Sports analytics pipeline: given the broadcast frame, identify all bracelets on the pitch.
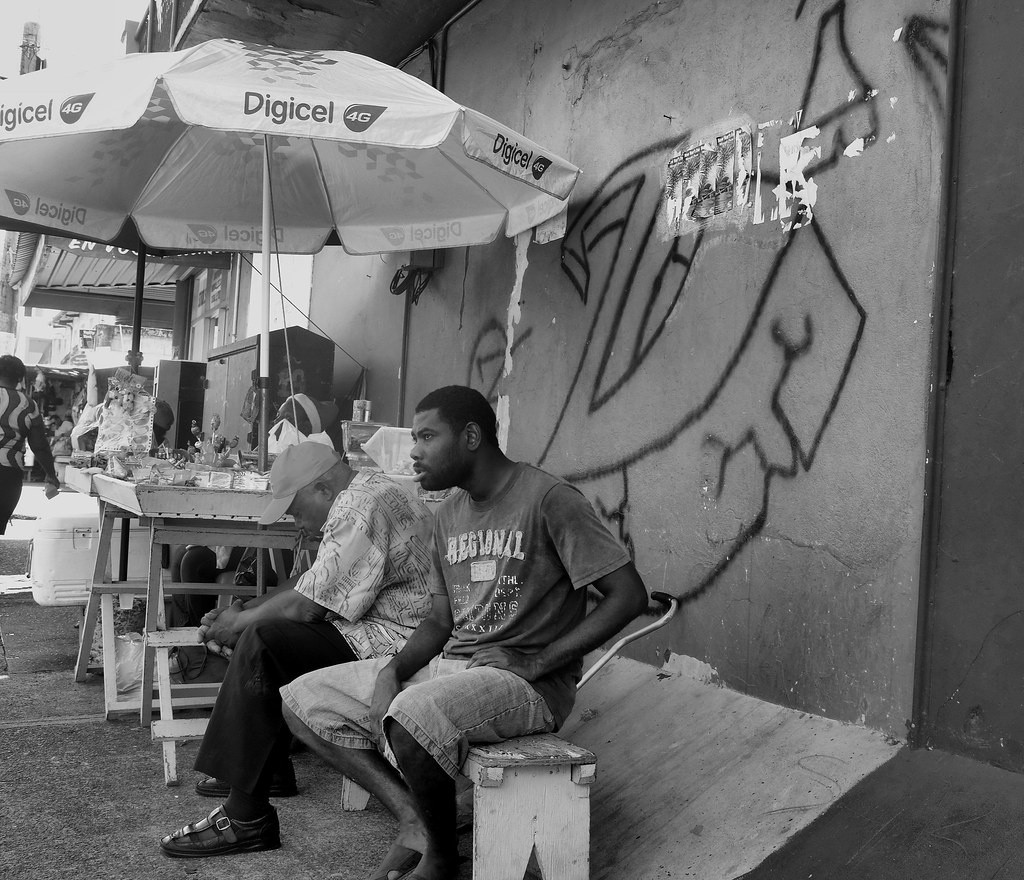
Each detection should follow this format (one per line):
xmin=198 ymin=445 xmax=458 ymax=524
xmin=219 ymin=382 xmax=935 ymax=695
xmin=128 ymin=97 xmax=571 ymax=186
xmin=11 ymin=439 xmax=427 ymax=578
xmin=239 ymin=602 xmax=246 ymax=612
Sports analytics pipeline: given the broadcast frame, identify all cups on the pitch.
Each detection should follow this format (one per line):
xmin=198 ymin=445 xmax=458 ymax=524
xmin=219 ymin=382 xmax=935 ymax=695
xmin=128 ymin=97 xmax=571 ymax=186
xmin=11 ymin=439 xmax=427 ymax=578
xmin=352 ymin=399 xmax=372 ymax=422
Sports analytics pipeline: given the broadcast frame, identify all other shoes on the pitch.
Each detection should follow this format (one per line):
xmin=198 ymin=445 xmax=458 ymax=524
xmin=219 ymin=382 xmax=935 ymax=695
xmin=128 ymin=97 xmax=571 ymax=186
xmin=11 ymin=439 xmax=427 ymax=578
xmin=366 ymin=835 xmax=459 ymax=880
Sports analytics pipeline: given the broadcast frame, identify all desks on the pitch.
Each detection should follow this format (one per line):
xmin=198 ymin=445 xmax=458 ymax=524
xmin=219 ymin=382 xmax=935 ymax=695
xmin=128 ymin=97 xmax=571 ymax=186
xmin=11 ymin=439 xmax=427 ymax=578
xmin=72 ymin=502 xmax=300 ymax=785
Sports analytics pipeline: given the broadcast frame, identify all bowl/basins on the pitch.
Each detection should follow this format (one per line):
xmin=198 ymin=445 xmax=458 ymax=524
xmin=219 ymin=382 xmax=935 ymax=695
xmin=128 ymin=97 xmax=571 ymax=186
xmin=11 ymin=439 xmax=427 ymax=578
xmin=341 ymin=421 xmax=389 ymax=473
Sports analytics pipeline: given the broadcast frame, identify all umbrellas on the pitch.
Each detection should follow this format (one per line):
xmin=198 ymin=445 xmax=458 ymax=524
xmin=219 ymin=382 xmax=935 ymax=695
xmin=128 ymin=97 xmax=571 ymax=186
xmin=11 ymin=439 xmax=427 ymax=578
xmin=0 ymin=37 xmax=582 ymax=598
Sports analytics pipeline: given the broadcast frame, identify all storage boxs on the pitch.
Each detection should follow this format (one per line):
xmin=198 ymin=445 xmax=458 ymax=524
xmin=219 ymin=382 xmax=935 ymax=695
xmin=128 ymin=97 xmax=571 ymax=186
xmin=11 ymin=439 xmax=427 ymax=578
xmin=93 ymin=472 xmax=296 ymax=524
xmin=30 ymin=492 xmax=171 ymax=607
xmin=64 ymin=464 xmax=99 ymax=497
xmin=340 ymin=420 xmax=391 ymax=452
xmin=345 ymin=453 xmax=378 ymax=471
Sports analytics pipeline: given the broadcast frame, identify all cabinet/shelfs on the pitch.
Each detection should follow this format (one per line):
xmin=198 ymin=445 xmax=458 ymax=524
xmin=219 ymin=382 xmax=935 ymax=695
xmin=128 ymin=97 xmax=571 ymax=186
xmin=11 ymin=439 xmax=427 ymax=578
xmin=201 ymin=326 xmax=336 ymax=456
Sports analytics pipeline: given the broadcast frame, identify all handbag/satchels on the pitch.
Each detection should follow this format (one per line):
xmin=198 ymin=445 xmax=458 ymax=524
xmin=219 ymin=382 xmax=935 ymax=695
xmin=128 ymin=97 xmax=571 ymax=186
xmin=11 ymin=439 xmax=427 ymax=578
xmin=115 ymin=632 xmax=159 ymax=702
xmin=267 ymin=419 xmax=336 ymax=456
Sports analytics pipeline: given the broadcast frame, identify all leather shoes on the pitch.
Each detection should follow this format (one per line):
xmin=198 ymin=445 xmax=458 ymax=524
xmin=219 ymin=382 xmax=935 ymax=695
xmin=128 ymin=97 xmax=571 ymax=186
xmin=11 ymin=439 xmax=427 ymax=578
xmin=159 ymin=803 xmax=281 ymax=858
xmin=194 ymin=757 xmax=298 ymax=796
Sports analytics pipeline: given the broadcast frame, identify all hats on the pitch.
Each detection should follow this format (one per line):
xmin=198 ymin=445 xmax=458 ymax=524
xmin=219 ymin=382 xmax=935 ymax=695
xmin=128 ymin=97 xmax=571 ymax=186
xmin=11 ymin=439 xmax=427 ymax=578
xmin=257 ymin=442 xmax=341 ymax=525
xmin=287 ymin=393 xmax=339 ymax=434
xmin=0 ymin=354 xmax=25 ymax=390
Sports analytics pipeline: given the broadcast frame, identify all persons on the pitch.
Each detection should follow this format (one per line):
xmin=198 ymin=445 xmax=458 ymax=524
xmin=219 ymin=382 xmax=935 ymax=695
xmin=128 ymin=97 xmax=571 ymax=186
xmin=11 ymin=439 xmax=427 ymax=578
xmin=161 ymin=393 xmax=436 ymax=859
xmin=0 ymin=354 xmax=73 ymax=535
xmin=279 ymin=385 xmax=648 ymax=880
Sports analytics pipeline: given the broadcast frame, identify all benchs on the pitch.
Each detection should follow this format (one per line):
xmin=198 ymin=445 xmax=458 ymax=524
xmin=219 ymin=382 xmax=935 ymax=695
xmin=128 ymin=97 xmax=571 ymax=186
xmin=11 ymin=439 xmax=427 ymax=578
xmin=340 ymin=733 xmax=596 ymax=880
